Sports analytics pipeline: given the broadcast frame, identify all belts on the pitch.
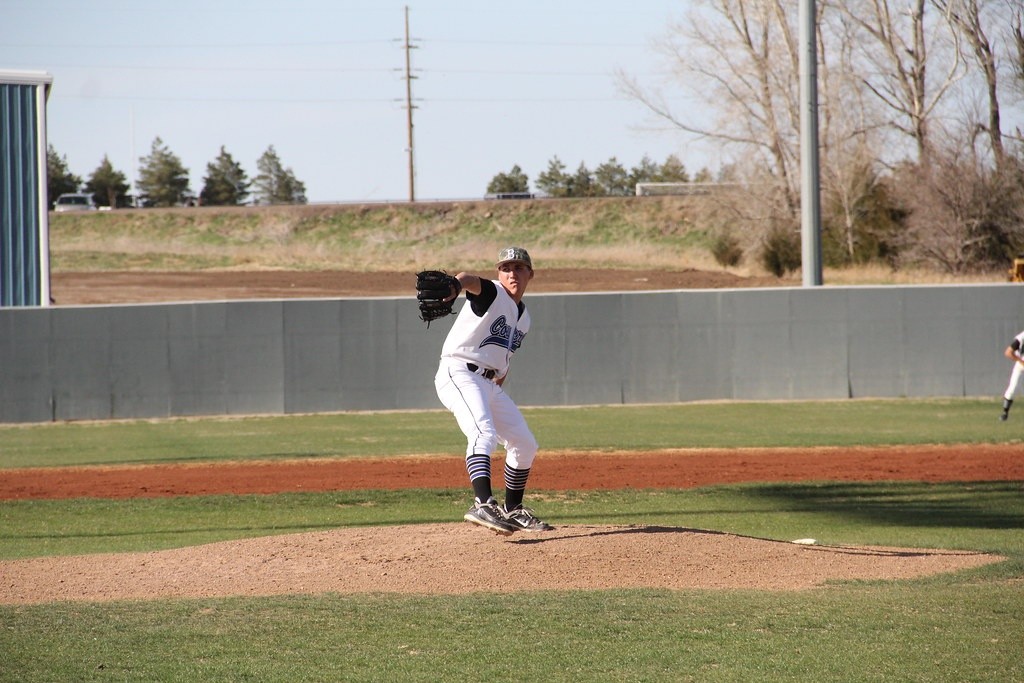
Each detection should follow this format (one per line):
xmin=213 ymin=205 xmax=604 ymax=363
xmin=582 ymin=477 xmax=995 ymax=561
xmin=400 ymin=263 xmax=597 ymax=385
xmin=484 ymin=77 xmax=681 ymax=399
xmin=467 ymin=363 xmax=495 ymax=379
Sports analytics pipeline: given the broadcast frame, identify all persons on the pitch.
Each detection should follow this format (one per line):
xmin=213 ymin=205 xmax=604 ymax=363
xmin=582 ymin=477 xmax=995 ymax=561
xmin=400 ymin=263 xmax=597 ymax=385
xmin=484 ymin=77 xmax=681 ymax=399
xmin=416 ymin=247 xmax=551 ymax=536
xmin=998 ymin=330 xmax=1024 ymax=422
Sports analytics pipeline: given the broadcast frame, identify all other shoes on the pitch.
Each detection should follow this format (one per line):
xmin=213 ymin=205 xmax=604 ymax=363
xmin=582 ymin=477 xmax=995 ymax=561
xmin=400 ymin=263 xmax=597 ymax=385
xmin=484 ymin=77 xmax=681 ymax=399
xmin=999 ymin=409 xmax=1008 ymax=419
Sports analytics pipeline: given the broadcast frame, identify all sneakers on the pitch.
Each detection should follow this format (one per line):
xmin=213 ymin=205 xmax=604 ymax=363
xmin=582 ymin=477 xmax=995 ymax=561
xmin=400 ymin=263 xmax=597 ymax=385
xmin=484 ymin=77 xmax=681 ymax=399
xmin=501 ymin=503 xmax=549 ymax=532
xmin=464 ymin=497 xmax=525 ymax=533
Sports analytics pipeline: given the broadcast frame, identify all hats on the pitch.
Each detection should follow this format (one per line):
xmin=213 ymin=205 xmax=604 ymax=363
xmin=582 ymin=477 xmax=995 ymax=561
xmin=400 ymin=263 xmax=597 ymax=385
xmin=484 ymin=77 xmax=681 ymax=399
xmin=495 ymin=247 xmax=531 ymax=269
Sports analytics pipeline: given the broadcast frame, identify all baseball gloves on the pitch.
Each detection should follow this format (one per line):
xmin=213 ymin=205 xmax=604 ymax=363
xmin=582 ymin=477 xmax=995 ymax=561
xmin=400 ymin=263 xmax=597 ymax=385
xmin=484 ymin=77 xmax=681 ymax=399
xmin=413 ymin=269 xmax=463 ymax=330
